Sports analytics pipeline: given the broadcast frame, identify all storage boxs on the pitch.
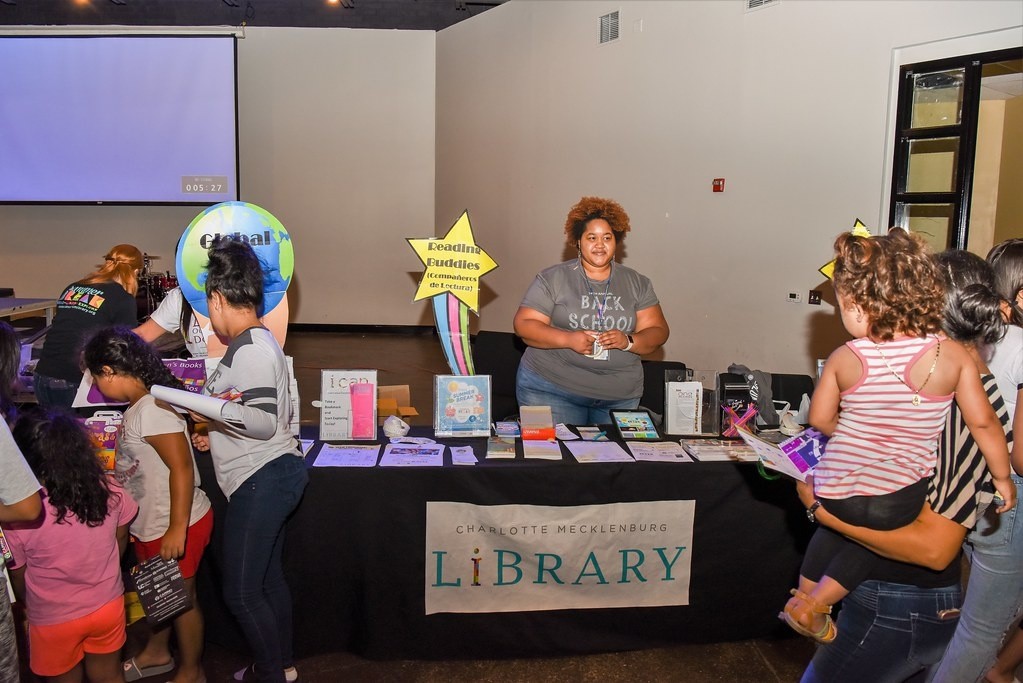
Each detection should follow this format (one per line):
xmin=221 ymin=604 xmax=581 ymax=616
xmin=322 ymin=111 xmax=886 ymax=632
xmin=377 ymin=397 xmax=419 ymax=425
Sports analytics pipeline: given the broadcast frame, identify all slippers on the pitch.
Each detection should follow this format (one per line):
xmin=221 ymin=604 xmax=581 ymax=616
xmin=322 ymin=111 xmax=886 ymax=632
xmin=122 ymin=656 xmax=175 ymax=682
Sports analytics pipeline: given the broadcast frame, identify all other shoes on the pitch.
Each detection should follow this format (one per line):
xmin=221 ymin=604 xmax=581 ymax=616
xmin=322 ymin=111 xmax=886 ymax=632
xmin=234 ymin=666 xmax=298 ymax=682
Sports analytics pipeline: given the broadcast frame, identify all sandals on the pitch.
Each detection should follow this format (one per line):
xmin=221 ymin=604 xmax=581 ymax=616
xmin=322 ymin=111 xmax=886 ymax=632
xmin=778 ymin=588 xmax=809 ymax=620
xmin=786 ymin=596 xmax=838 ymax=644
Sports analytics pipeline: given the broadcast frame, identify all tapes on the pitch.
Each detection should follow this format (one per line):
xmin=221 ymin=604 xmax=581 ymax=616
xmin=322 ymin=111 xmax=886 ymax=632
xmin=583 ymin=334 xmax=603 ymax=358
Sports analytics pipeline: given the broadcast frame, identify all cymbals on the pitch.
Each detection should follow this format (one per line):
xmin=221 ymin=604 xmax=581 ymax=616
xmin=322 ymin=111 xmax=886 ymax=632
xmin=141 ymin=255 xmax=162 ymax=260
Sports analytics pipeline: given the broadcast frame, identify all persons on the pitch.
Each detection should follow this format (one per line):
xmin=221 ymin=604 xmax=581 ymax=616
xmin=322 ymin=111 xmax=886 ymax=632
xmin=1 ymin=404 xmax=138 ymax=683
xmin=0 ymin=319 xmax=44 ymax=683
xmin=924 ymin=238 xmax=1023 ymax=683
xmin=797 ymin=250 xmax=1014 ymax=683
xmin=512 ymin=196 xmax=670 ymax=429
xmin=129 ymin=238 xmax=208 ymax=358
xmin=187 ymin=234 xmax=310 ymax=683
xmin=85 ymin=326 xmax=213 ymax=683
xmin=33 ymin=244 xmax=144 ymax=422
xmin=778 ymin=226 xmax=1017 ymax=644
xmin=985 ymin=619 xmax=1023 ymax=683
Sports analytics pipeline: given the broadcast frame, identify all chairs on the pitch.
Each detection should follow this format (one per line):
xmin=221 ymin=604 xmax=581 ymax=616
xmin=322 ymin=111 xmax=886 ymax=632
xmin=638 ymin=361 xmax=687 ymax=424
xmin=473 ymin=330 xmax=528 ymax=422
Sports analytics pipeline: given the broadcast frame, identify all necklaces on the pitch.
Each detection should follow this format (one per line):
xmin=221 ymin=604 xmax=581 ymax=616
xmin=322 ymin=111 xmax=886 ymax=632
xmin=875 ymin=332 xmax=941 ymax=407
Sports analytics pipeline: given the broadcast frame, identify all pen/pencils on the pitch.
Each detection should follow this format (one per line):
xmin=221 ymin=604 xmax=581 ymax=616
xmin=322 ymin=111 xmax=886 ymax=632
xmin=721 ymin=401 xmax=760 ymax=437
xmin=592 ymin=432 xmax=606 ymax=440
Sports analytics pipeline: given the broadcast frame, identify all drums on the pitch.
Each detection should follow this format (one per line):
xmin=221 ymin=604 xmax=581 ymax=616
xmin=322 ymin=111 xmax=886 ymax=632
xmin=139 ymin=284 xmax=157 ymax=313
xmin=149 ymin=272 xmax=164 ymax=291
xmin=160 ymin=275 xmax=177 ymax=290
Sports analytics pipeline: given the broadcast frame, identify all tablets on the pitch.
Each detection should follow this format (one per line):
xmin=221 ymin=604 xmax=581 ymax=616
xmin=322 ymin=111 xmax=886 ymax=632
xmin=609 ymin=408 xmax=661 ymax=441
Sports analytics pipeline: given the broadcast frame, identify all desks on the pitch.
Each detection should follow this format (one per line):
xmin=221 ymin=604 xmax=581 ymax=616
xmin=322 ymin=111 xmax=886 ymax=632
xmin=172 ymin=423 xmax=813 ymax=658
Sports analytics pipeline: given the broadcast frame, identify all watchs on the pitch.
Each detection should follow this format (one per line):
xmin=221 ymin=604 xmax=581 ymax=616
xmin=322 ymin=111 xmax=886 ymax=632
xmin=807 ymin=501 xmax=822 ymax=523
xmin=622 ymin=334 xmax=634 ymax=352
